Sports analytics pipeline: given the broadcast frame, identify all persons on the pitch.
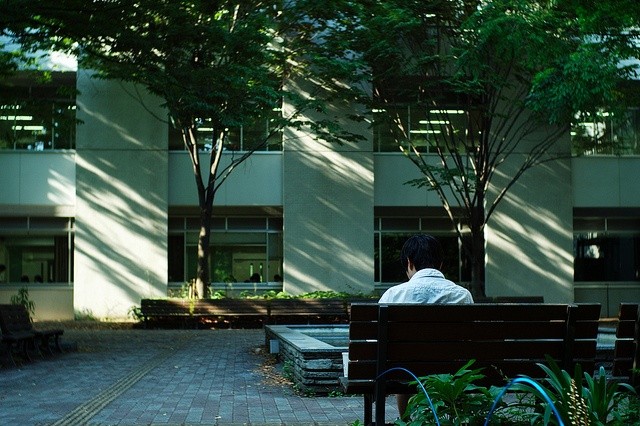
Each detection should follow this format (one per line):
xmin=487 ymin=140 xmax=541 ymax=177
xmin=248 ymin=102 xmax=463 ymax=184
xmin=274 ymin=274 xmax=281 ymax=283
xmin=34 ymin=275 xmax=43 ymax=281
xmin=250 ymin=273 xmax=261 ymax=282
xmin=377 ymin=233 xmax=475 ymax=423
xmin=22 ymin=275 xmax=30 ymax=282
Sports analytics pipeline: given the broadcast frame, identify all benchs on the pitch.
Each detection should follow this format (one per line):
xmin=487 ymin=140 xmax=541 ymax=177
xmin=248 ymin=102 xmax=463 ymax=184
xmin=0 ymin=303 xmax=64 ymax=367
xmin=270 ymin=299 xmax=348 ymax=324
xmin=611 ymin=303 xmax=639 ymax=393
xmin=192 ymin=299 xmax=269 ymax=329
xmin=133 ymin=299 xmax=190 ymax=328
xmin=344 ymin=304 xmax=602 ymax=425
